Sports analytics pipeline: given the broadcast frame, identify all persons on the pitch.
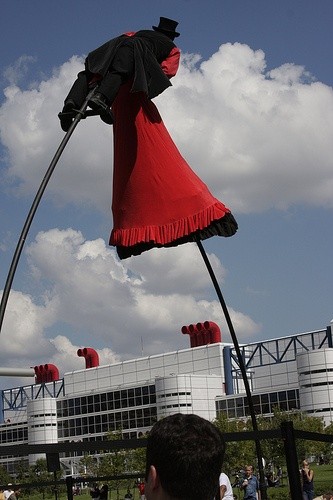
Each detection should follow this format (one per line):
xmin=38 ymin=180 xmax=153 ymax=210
xmin=235 ymin=464 xmax=284 ymax=500
xmin=214 ymin=470 xmax=234 ymax=500
xmin=59 ymin=17 xmax=180 ymax=131
xmin=72 ymin=482 xmax=145 ymax=500
xmin=0 ymin=482 xmax=22 ymax=500
xmin=301 ymin=459 xmax=314 ymax=500
xmin=142 ymin=413 xmax=225 ymax=500
